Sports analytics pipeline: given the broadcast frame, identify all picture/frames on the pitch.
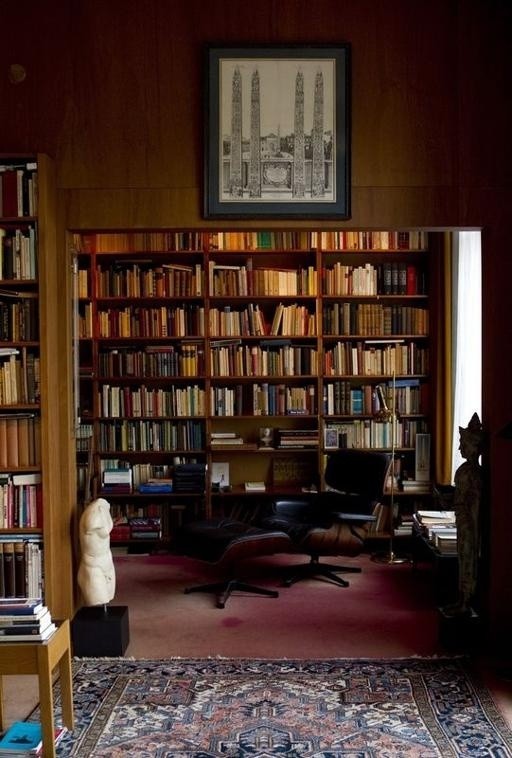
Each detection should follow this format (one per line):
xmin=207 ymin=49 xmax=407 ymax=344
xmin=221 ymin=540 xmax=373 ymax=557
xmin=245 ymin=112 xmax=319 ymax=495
xmin=198 ymin=35 xmax=352 ymax=219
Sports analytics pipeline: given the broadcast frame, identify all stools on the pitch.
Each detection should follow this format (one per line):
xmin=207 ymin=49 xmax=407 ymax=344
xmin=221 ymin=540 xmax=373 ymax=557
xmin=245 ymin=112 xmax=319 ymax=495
xmin=172 ymin=518 xmax=292 ymax=609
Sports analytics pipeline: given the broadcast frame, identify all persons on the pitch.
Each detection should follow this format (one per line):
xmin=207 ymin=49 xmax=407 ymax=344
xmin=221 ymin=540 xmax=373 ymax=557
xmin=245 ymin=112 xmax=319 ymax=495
xmin=77 ymin=497 xmax=117 ymax=607
xmin=454 ymin=414 xmax=486 ymax=613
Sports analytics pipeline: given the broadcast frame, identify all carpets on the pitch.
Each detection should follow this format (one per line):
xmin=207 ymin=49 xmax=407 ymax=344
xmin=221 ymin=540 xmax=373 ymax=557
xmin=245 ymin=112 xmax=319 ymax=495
xmin=23 ymin=657 xmax=512 ymax=758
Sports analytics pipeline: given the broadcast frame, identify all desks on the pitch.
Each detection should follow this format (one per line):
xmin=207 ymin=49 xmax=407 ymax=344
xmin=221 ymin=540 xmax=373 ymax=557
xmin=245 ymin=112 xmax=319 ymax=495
xmin=0 ymin=620 xmax=77 ymax=758
xmin=411 ymin=525 xmax=459 ymax=589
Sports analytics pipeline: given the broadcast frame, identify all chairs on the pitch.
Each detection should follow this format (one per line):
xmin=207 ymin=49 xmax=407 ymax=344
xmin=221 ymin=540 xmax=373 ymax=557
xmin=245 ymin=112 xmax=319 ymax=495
xmin=260 ymin=450 xmax=388 ymax=589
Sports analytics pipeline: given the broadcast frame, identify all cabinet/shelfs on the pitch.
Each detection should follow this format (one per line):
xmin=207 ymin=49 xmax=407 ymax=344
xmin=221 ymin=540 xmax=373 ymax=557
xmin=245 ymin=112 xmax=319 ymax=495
xmin=0 ymin=152 xmax=76 ymax=621
xmin=75 ymin=224 xmax=450 ymax=549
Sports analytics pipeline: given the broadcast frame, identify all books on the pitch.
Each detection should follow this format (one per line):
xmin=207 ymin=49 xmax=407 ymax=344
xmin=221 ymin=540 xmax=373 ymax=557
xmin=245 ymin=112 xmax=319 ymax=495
xmin=74 ymin=229 xmax=204 ymax=526
xmin=0 ymin=721 xmax=68 ymax=757
xmin=392 ymin=511 xmax=458 ymax=554
xmin=0 ymin=164 xmax=58 ymax=643
xmin=207 ymin=230 xmax=318 ymax=491
xmin=318 ymin=230 xmax=430 ymax=492
